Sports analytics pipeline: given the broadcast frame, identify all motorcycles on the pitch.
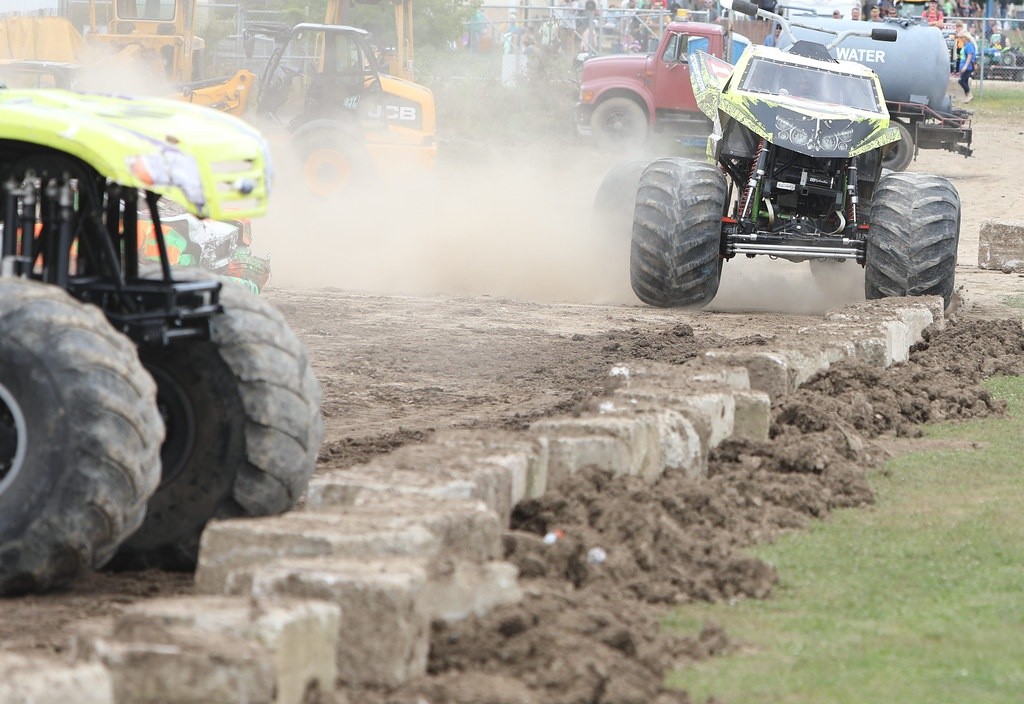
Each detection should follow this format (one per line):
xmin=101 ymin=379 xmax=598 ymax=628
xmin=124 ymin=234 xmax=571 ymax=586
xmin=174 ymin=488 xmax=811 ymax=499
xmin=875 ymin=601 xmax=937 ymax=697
xmin=630 ymin=0 xmax=962 ymax=317
xmin=0 ymin=137 xmax=321 ymax=597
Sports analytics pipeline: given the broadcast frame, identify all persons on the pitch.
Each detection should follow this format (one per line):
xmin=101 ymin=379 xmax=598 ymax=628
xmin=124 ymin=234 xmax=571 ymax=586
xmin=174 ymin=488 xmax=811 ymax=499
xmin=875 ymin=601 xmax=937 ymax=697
xmin=867 ymin=6 xmax=884 ymax=22
xmin=763 ymin=18 xmax=788 ymax=47
xmin=460 ymin=0 xmax=720 ymax=60
xmin=888 ymin=8 xmax=897 ymax=16
xmin=851 ymin=8 xmax=860 ymax=21
xmin=794 ymin=72 xmax=815 ymax=97
xmin=922 ymin=0 xmax=1024 ymax=77
xmin=958 ymin=32 xmax=977 ymax=103
xmin=833 ymin=10 xmax=841 ymax=19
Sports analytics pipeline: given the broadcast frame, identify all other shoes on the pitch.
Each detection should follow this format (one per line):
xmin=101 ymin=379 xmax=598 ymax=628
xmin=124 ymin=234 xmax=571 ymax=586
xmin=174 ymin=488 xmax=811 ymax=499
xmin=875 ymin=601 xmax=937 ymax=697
xmin=964 ymin=94 xmax=973 ymax=103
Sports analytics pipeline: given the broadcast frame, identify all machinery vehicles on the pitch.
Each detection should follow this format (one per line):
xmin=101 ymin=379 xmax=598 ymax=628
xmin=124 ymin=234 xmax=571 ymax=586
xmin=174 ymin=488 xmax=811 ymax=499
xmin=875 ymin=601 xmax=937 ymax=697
xmin=0 ymin=1 xmax=450 ymax=187
xmin=571 ymin=8 xmax=973 ymax=174
